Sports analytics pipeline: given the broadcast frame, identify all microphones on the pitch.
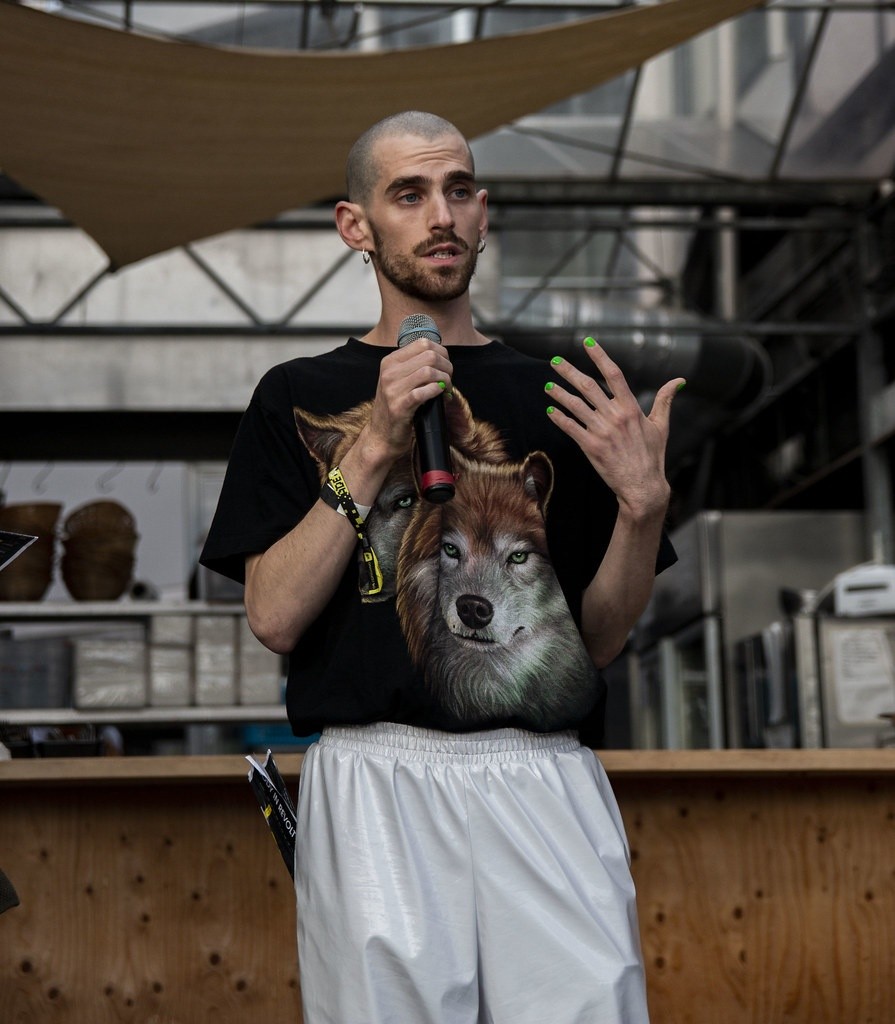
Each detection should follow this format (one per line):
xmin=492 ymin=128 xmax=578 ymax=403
xmin=396 ymin=312 xmax=456 ymax=504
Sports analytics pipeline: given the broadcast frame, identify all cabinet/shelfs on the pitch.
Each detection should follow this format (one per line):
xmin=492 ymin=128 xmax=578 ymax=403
xmin=0 ymin=599 xmax=286 ymax=724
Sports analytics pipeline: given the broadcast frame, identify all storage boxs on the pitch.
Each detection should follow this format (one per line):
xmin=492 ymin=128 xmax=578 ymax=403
xmin=72 ymin=607 xmax=281 ymax=710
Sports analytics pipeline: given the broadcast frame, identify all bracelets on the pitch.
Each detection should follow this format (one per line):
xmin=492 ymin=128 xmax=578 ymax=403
xmin=321 ymin=485 xmax=371 ymax=526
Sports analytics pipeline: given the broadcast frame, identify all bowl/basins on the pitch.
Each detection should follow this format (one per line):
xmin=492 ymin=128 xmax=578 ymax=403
xmin=0 ymin=499 xmax=139 ymax=603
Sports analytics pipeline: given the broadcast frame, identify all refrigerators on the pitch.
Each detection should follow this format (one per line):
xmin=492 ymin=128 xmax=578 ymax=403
xmin=628 ymin=511 xmax=860 ymax=750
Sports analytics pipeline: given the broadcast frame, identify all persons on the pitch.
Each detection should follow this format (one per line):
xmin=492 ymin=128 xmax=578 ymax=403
xmin=199 ymin=111 xmax=687 ymax=1024
